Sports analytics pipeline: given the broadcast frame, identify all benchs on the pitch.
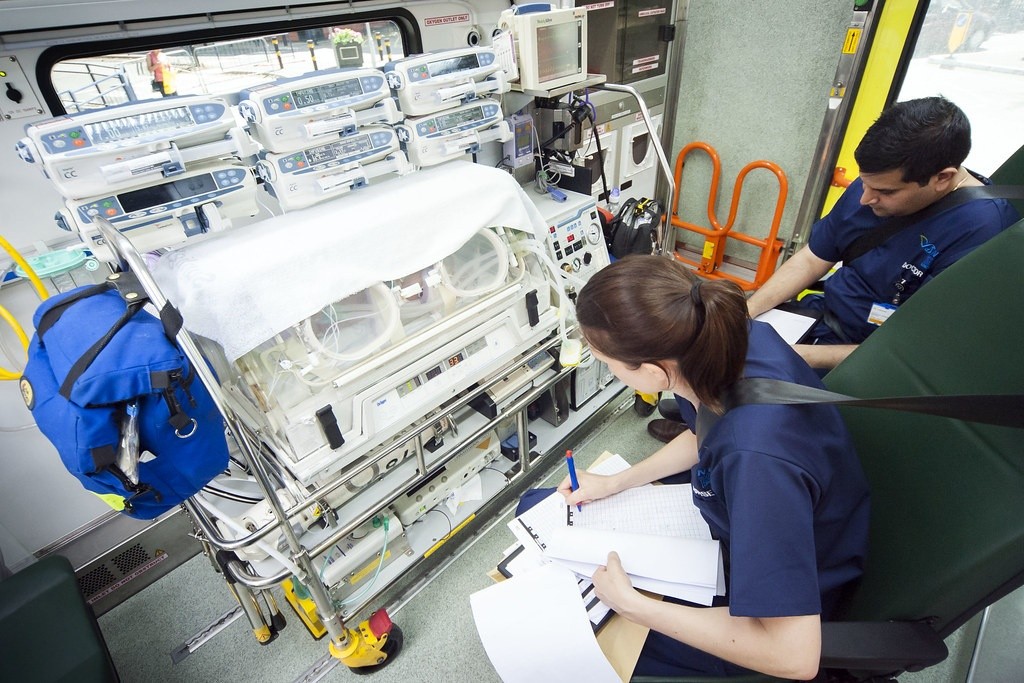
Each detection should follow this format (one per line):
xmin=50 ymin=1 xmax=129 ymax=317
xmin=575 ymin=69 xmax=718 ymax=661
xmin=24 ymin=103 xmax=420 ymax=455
xmin=0 ymin=554 xmax=121 ymax=683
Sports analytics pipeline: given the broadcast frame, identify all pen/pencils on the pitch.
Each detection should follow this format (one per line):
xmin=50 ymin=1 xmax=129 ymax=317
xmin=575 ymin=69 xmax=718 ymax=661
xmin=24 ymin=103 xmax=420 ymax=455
xmin=566 ymin=449 xmax=581 ymax=512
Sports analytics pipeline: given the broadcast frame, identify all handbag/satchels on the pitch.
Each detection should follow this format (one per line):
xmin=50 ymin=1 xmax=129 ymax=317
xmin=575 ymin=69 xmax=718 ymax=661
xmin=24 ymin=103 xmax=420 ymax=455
xmin=18 ymin=279 xmax=230 ymax=519
xmin=607 ymin=197 xmax=662 ymax=259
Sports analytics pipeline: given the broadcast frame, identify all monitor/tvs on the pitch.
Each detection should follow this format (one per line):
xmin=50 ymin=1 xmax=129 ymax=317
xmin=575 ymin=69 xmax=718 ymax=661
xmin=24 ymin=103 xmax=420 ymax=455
xmin=499 ymin=6 xmax=588 ymax=93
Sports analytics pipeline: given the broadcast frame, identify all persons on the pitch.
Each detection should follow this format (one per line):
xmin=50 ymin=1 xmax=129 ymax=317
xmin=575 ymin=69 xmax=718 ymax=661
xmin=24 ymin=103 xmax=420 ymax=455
xmin=648 ymin=95 xmax=1016 ymax=444
xmin=146 ymin=49 xmax=178 ymax=97
xmin=557 ymin=254 xmax=868 ymax=680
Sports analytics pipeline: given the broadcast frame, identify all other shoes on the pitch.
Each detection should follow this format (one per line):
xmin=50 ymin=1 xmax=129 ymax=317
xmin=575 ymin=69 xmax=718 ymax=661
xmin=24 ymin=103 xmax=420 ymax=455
xmin=658 ymin=398 xmax=694 ymax=422
xmin=648 ymin=419 xmax=689 ymax=443
